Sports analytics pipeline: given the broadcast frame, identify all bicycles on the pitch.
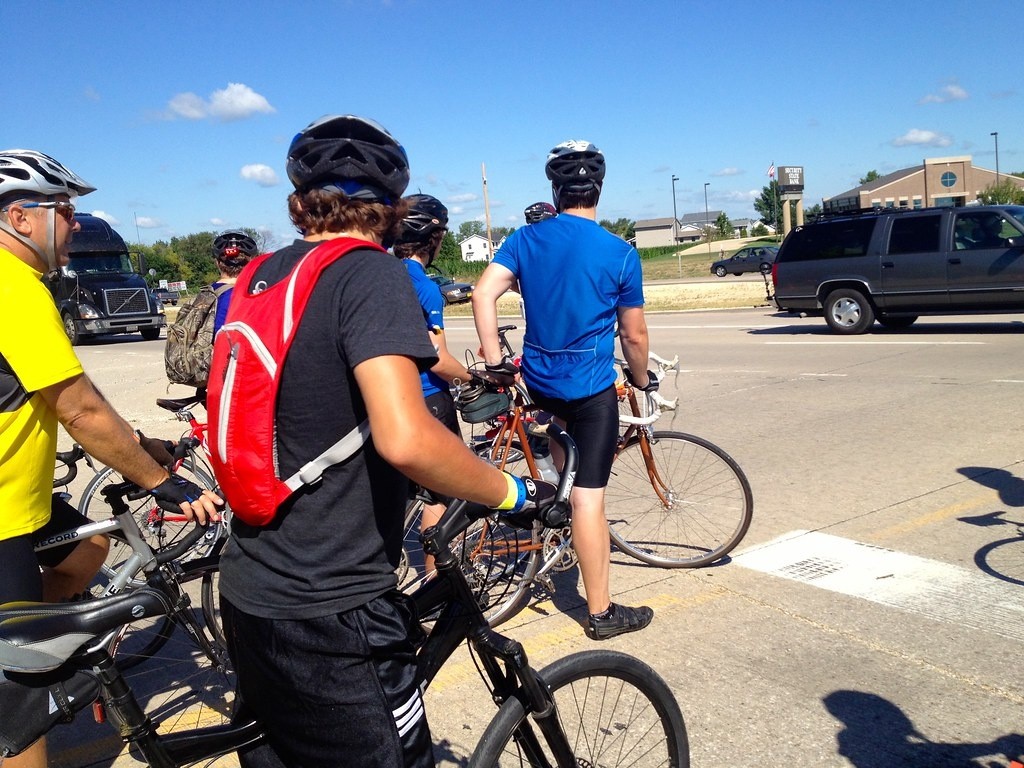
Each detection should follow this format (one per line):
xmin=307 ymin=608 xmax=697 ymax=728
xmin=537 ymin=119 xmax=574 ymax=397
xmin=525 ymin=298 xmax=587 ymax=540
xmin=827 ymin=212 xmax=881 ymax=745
xmin=3 ymin=324 xmax=687 ymax=768
xmin=434 ymin=348 xmax=748 ymax=570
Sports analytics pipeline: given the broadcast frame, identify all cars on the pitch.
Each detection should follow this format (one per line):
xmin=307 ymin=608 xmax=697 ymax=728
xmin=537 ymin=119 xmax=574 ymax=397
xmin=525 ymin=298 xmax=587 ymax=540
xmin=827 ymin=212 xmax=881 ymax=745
xmin=427 ymin=273 xmax=471 ymax=307
xmin=709 ymin=246 xmax=781 ymax=277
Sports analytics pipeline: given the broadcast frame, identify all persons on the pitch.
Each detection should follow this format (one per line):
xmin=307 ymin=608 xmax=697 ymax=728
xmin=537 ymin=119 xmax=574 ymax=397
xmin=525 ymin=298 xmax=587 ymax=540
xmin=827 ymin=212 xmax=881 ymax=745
xmin=470 ymin=139 xmax=658 ymax=642
xmin=207 ymin=115 xmax=574 ymax=768
xmin=978 ymin=213 xmax=1009 ymax=247
xmin=0 ymin=149 xmax=223 ymax=768
xmin=509 ymin=201 xmax=555 ymax=438
xmin=196 ymin=229 xmax=260 ymax=411
xmin=392 ymin=194 xmax=490 ymax=619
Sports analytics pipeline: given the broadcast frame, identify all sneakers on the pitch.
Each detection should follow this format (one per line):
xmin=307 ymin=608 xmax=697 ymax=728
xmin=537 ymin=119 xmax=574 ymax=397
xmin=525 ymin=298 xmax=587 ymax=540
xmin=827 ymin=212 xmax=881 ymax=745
xmin=426 ymin=590 xmax=490 ymax=617
xmin=588 ymin=601 xmax=654 ymax=640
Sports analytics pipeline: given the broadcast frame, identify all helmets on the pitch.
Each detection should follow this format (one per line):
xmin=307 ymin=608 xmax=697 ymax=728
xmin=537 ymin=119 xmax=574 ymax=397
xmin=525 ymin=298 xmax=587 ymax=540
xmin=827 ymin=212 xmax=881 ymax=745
xmin=524 ymin=202 xmax=557 ymax=224
xmin=545 ymin=140 xmax=606 ymax=180
xmin=393 ymin=194 xmax=448 ymax=242
xmin=211 ymin=228 xmax=258 ymax=259
xmin=0 ymin=149 xmax=98 ymax=196
xmin=286 ymin=115 xmax=410 ymax=198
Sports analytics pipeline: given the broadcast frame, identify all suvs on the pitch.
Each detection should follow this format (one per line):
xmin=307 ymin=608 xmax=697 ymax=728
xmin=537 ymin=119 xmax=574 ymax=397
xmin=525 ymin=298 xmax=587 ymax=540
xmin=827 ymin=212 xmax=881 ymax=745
xmin=771 ymin=207 xmax=1022 ymax=334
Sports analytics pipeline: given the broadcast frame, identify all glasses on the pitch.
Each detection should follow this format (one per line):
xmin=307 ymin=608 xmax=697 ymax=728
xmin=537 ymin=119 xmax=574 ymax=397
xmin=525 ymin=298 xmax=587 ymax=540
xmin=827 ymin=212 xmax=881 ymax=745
xmin=3 ymin=202 xmax=75 ymax=221
xmin=438 ymin=226 xmax=447 ymax=236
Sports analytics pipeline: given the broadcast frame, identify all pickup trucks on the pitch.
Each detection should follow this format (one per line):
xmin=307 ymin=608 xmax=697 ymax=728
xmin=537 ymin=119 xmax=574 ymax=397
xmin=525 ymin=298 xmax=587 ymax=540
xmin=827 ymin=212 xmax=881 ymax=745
xmin=151 ymin=288 xmax=179 ymax=306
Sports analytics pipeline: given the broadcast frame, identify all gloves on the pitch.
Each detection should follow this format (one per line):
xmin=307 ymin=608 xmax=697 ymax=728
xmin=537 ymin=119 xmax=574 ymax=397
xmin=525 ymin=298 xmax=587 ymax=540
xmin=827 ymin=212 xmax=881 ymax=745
xmin=503 ymin=475 xmax=572 ymax=532
xmin=469 ymin=373 xmax=498 ymax=392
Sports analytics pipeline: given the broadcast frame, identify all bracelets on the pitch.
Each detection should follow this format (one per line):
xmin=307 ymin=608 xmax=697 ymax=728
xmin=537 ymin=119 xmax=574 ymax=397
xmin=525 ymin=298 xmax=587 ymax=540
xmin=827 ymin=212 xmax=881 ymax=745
xmin=487 ymin=469 xmax=526 ymax=517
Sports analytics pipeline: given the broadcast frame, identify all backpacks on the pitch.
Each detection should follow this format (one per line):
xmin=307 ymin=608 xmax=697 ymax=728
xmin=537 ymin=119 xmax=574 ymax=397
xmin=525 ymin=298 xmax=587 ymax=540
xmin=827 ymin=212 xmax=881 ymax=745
xmin=207 ymin=238 xmax=387 ymax=524
xmin=165 ymin=281 xmax=235 ymax=394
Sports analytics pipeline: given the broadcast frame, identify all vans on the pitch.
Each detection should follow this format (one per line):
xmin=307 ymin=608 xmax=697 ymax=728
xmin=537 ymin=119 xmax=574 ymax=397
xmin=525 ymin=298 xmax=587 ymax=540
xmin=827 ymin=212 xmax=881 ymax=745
xmin=42 ymin=212 xmax=166 ymax=347
